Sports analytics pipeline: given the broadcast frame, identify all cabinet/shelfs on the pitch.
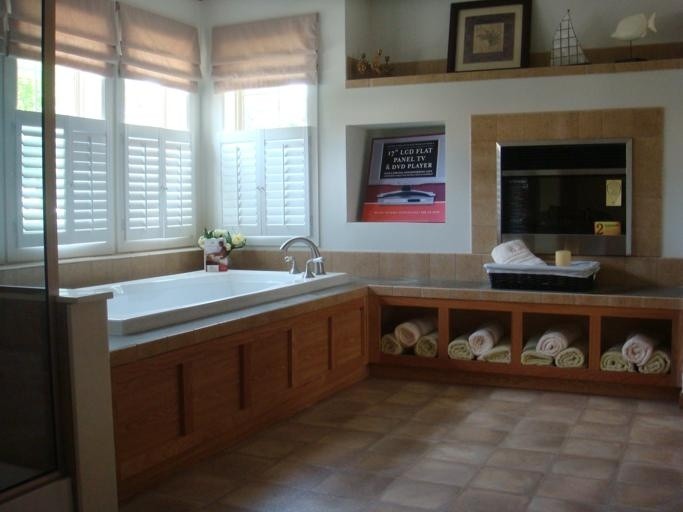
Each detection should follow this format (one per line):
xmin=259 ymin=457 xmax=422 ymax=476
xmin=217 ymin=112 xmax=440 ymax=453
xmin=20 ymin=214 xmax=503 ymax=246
xmin=367 ymin=283 xmax=682 ymax=403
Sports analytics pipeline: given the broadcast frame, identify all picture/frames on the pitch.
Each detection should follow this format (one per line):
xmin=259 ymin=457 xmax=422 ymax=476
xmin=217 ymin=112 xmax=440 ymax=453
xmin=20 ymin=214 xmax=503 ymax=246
xmin=444 ymin=0 xmax=531 ymax=74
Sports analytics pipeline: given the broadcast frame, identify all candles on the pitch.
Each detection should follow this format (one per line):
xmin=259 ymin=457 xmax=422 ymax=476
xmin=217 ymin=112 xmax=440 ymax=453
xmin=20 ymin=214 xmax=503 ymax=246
xmin=555 ymin=250 xmax=572 ymax=266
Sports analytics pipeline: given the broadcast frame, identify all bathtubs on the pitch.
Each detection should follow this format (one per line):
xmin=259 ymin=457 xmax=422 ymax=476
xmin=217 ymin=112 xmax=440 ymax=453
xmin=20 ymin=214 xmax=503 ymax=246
xmin=58 ymin=269 xmax=350 ymax=337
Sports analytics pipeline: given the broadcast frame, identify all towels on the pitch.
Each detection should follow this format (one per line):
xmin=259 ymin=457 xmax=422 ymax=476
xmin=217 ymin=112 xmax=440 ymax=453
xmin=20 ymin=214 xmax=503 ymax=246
xmin=491 ymin=239 xmax=547 ymax=269
xmin=381 ymin=313 xmax=672 ymax=377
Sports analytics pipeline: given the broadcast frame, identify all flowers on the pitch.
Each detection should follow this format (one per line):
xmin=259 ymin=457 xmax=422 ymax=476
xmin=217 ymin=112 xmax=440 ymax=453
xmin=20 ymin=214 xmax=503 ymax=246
xmin=196 ymin=226 xmax=246 ymax=252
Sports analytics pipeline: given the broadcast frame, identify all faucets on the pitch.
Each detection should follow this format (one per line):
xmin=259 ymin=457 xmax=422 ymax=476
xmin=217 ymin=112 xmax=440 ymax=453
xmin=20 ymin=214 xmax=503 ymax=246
xmin=280 ymin=236 xmax=326 ymax=275
